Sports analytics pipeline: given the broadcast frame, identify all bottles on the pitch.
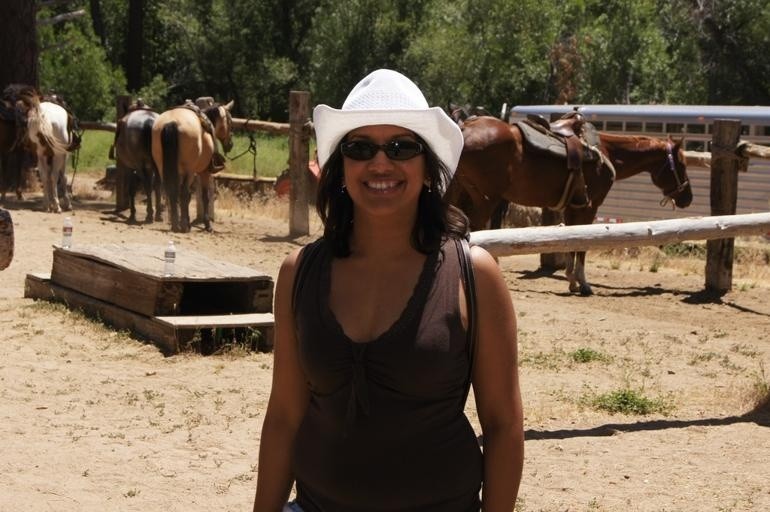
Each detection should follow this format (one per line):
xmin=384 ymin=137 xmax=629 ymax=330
xmin=62 ymin=215 xmax=73 ymax=250
xmin=165 ymin=242 xmax=176 ymax=278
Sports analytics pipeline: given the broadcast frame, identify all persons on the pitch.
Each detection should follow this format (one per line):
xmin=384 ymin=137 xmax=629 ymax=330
xmin=251 ymin=64 xmax=524 ymax=511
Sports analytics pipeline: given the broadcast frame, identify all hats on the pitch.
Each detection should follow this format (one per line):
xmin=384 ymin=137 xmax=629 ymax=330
xmin=313 ymin=68 xmax=465 ymax=199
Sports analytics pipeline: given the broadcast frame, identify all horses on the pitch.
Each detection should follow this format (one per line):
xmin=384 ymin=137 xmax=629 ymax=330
xmin=455 ymin=115 xmax=692 ymax=297
xmin=116 ymin=108 xmax=163 ymax=225
xmin=151 ymin=99 xmax=235 ymax=233
xmin=443 ymin=106 xmax=586 ymax=277
xmin=27 ymin=101 xmax=76 ymax=213
xmin=0 ymin=99 xmax=40 ymax=204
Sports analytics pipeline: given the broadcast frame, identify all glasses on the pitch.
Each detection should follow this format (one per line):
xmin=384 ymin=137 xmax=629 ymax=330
xmin=340 ymin=140 xmax=423 ymax=161
